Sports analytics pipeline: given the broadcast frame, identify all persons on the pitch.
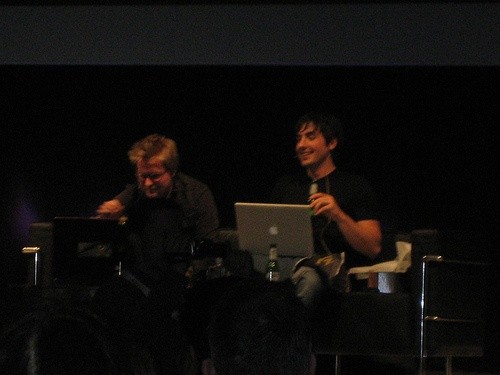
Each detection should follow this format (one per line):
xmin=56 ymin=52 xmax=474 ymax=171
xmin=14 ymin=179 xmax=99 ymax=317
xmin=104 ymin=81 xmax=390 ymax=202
xmin=0 ymin=278 xmax=316 ymax=375
xmin=272 ymin=111 xmax=381 ymax=271
xmin=92 ymin=136 xmax=221 ymax=277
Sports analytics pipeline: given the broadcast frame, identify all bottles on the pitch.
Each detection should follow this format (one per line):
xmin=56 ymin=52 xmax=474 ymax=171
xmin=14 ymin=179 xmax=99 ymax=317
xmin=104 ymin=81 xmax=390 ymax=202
xmin=265 ymin=244 xmax=281 ymax=282
xmin=307 ymin=179 xmax=319 ymax=216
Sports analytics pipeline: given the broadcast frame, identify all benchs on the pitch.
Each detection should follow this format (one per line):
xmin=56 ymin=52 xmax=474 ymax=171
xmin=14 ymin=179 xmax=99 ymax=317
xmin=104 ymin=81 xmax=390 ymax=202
xmin=22 ymin=221 xmax=489 ymax=375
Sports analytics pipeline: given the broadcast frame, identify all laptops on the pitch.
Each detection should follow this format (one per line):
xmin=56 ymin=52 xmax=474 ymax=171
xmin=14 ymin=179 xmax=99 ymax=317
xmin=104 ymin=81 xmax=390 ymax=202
xmin=235 ymin=202 xmax=314 ymax=257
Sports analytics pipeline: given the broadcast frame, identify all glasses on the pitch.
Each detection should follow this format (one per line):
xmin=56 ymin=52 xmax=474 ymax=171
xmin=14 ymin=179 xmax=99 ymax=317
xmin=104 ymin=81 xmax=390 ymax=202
xmin=136 ymin=169 xmax=168 ymax=182
